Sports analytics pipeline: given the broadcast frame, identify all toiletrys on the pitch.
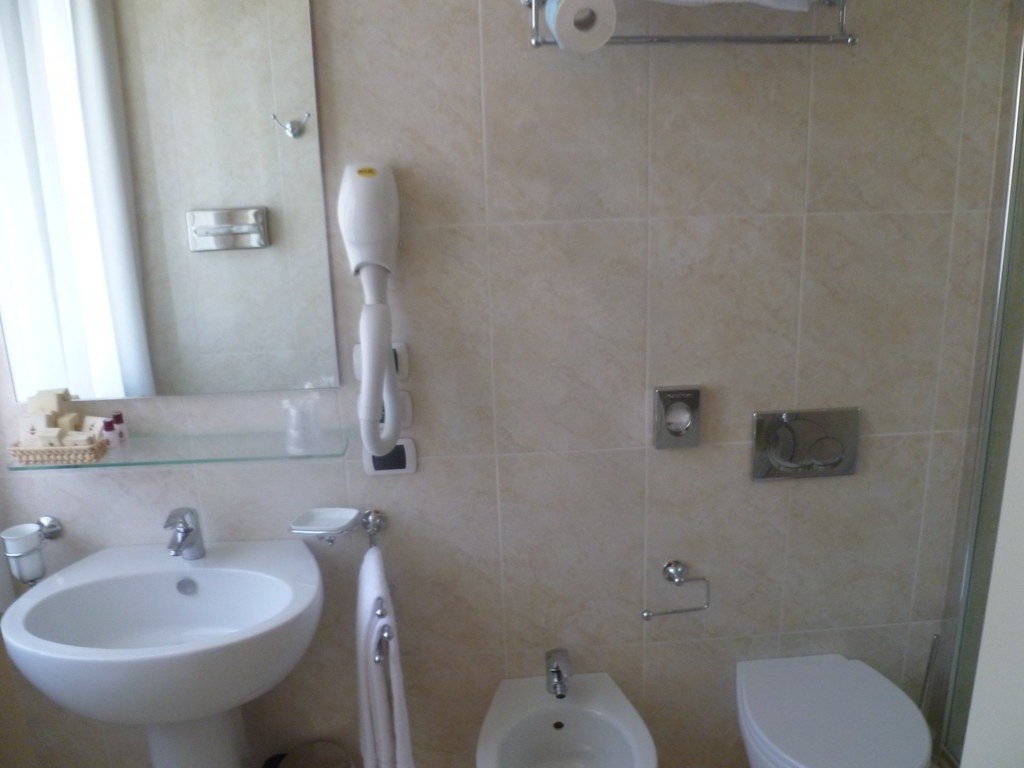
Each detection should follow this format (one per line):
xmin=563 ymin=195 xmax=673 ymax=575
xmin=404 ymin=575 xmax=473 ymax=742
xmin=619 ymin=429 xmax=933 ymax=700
xmin=102 ymin=412 xmax=131 ymax=459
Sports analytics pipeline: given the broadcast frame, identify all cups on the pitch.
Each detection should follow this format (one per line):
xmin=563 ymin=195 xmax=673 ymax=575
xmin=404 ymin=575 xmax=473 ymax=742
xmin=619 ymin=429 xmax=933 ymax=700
xmin=0 ymin=524 xmax=45 ymax=582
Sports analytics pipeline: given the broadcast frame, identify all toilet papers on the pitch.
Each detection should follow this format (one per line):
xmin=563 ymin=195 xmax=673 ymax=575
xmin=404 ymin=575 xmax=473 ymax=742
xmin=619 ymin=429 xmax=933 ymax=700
xmin=543 ymin=0 xmax=618 ymax=55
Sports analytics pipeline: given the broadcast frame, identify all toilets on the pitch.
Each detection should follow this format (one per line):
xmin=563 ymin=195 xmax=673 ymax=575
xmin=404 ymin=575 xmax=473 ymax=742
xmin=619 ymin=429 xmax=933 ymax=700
xmin=735 ymin=652 xmax=933 ymax=767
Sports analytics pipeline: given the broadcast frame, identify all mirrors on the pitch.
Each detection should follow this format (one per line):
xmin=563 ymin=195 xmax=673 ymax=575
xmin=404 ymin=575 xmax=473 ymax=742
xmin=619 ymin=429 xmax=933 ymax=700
xmin=0 ymin=0 xmax=342 ymax=404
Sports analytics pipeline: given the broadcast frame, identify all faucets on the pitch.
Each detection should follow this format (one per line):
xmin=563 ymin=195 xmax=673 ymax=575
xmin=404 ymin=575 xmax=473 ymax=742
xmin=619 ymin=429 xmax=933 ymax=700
xmin=544 ymin=647 xmax=576 ymax=700
xmin=161 ymin=506 xmax=208 ymax=562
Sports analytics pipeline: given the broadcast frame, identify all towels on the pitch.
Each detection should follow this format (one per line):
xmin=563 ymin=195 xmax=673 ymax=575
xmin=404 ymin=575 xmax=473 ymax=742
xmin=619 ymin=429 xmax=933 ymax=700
xmin=355 ymin=545 xmax=414 ymax=767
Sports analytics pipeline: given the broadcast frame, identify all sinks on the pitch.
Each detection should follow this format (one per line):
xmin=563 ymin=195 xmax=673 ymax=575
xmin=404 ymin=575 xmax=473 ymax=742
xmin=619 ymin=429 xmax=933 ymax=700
xmin=0 ymin=537 xmax=324 ymax=725
xmin=475 ymin=671 xmax=659 ymax=768
